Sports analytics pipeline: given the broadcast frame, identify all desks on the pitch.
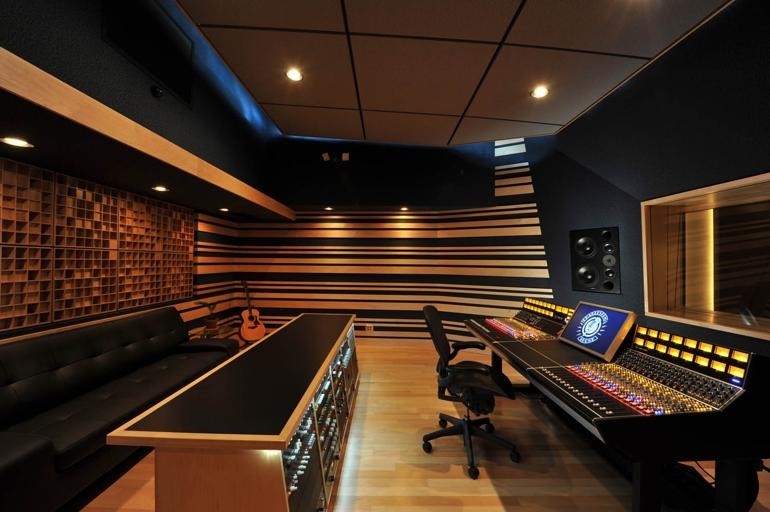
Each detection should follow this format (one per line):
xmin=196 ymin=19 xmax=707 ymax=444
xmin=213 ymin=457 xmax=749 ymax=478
xmin=107 ymin=313 xmax=360 ymax=512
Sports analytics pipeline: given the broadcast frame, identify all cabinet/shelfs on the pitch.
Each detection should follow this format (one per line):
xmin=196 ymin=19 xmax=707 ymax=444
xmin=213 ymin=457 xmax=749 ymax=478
xmin=1 ymin=157 xmax=194 ymax=330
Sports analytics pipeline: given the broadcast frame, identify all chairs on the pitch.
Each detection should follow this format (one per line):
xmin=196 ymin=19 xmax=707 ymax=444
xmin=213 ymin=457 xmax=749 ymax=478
xmin=423 ymin=305 xmax=520 ymax=479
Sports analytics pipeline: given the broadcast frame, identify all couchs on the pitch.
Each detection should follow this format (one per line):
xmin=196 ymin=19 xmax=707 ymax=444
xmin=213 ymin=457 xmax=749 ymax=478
xmin=0 ymin=306 xmax=240 ymax=511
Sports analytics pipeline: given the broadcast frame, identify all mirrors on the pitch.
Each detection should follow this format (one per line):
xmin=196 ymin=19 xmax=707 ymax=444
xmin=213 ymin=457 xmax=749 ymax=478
xmin=641 ymin=172 xmax=769 ymax=341
